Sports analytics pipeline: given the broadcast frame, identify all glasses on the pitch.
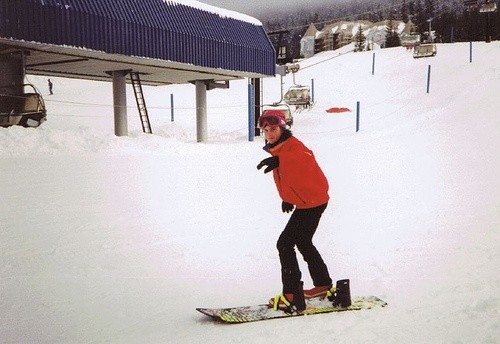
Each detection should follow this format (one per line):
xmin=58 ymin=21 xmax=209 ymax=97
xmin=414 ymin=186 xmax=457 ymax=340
xmin=257 ymin=114 xmax=285 ymax=129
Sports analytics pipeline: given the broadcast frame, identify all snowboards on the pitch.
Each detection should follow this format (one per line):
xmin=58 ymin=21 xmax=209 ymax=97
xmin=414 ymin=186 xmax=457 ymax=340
xmin=196 ymin=295 xmax=387 ymax=323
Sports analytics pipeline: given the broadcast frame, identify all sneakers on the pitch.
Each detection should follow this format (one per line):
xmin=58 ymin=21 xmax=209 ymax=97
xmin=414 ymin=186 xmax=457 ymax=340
xmin=269 ymin=294 xmax=297 ymax=310
xmin=303 ymin=283 xmax=332 ymax=298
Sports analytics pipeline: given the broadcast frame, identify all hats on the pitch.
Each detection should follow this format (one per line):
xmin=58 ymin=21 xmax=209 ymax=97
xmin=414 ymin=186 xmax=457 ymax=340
xmin=262 ymin=110 xmax=285 ymax=127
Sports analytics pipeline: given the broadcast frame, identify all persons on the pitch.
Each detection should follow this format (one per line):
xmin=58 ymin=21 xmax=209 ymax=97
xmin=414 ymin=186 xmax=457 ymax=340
xmin=255 ymin=108 xmax=334 ymax=311
xmin=47 ymin=79 xmax=53 ymax=95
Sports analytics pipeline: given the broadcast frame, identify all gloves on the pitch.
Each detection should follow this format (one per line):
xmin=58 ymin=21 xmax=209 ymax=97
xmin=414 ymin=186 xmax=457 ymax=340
xmin=281 ymin=202 xmax=294 ymax=213
xmin=257 ymin=156 xmax=278 ymax=173
